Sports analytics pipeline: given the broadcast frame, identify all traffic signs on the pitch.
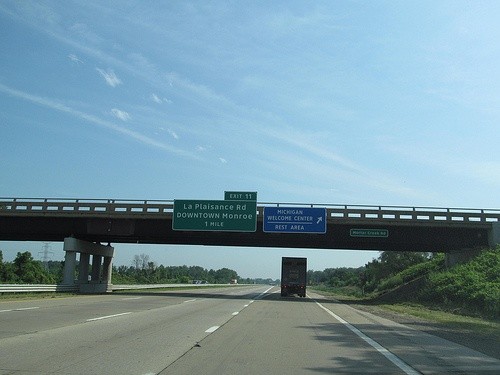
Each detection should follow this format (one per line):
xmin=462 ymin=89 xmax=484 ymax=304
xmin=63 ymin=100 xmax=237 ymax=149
xmin=262 ymin=207 xmax=327 ymax=233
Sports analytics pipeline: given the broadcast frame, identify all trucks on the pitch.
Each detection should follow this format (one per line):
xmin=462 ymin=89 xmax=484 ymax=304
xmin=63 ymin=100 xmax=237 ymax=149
xmin=231 ymin=279 xmax=237 ymax=284
xmin=281 ymin=257 xmax=307 ymax=297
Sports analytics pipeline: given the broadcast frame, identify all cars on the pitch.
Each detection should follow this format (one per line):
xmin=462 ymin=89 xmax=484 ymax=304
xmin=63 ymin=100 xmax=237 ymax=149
xmin=193 ymin=280 xmax=209 ymax=285
